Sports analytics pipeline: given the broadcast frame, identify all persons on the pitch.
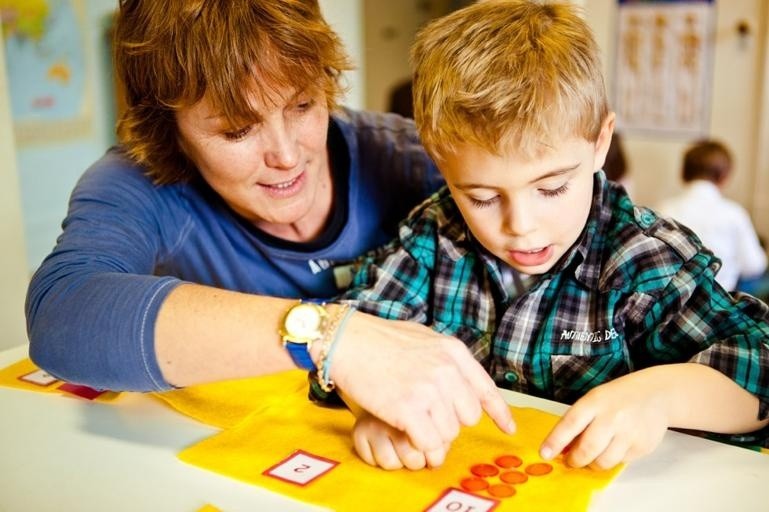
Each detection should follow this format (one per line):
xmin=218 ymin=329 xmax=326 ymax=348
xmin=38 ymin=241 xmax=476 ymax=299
xmin=329 ymin=0 xmax=768 ymax=471
xmin=650 ymin=135 xmax=768 ymax=296
xmin=22 ymin=0 xmax=523 ymax=445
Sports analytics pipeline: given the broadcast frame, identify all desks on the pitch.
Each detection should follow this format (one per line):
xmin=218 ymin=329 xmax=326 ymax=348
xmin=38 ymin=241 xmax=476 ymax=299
xmin=0 ymin=346 xmax=769 ymax=512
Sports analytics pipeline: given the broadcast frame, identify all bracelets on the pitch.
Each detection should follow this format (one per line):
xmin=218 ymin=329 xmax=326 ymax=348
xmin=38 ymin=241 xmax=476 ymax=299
xmin=322 ymin=306 xmax=360 ymax=386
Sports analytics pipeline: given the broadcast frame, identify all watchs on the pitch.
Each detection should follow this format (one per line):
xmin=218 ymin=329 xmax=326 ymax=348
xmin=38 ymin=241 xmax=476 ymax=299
xmin=278 ymin=296 xmax=327 ymax=374
